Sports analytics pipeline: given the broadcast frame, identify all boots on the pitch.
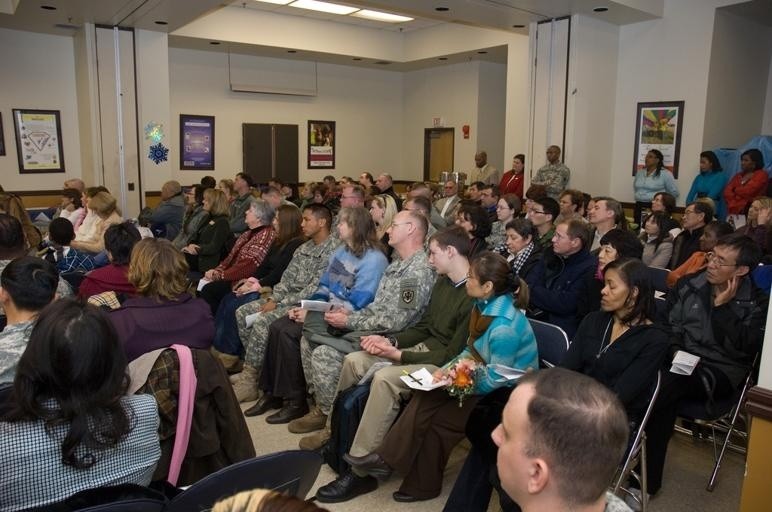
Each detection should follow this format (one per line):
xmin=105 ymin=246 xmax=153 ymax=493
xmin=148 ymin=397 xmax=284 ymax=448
xmin=229 ymin=364 xmax=260 ymax=403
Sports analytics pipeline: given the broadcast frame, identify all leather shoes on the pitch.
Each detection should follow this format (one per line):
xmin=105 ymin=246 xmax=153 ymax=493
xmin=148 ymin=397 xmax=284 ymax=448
xmin=393 ymin=490 xmax=419 ymax=504
xmin=315 ymin=468 xmax=372 ymax=504
xmin=266 ymin=404 xmax=311 ymax=425
xmin=243 ymin=393 xmax=284 ymax=417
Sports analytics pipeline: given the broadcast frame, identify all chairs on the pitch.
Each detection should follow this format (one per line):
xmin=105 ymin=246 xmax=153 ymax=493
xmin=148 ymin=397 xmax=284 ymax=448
xmin=60 ymin=270 xmax=83 ymax=295
xmin=168 ymin=451 xmax=324 ymax=511
xmin=694 ymin=349 xmax=757 ymax=493
xmin=1 ymin=499 xmax=165 ymax=512
xmin=647 ymin=265 xmax=671 ymax=324
xmin=752 ymin=264 xmax=772 ymax=297
xmin=525 ymin=319 xmax=570 ymax=370
xmin=442 ymin=438 xmax=473 ymax=478
xmin=610 ymin=370 xmax=662 ymax=511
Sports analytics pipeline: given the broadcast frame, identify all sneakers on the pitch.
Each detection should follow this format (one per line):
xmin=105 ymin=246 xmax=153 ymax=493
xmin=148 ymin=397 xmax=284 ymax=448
xmin=299 ymin=427 xmax=331 ymax=453
xmin=287 ymin=405 xmax=328 ymax=434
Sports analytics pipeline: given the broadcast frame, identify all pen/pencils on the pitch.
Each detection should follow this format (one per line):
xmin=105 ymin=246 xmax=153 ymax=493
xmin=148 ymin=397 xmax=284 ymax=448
xmin=330 ymin=304 xmax=334 ymax=313
xmin=402 ymin=370 xmax=423 ymax=385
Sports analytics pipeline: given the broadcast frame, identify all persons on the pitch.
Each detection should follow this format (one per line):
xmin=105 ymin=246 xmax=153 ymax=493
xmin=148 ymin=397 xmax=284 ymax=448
xmin=632 ymin=149 xmax=680 ymax=228
xmin=320 ymin=125 xmax=332 ymax=145
xmin=470 ymin=151 xmax=499 ymax=186
xmin=685 ymin=151 xmax=730 ymax=220
xmin=723 ymin=147 xmax=772 ymax=218
xmin=529 ymin=144 xmax=572 ymax=203
xmin=497 ymin=154 xmax=525 ymax=198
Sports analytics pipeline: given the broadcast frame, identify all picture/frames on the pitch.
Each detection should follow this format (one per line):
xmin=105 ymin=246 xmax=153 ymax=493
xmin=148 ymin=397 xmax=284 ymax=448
xmin=630 ymin=98 xmax=687 ymax=180
xmin=307 ymin=118 xmax=336 ymax=170
xmin=11 ymin=107 xmax=67 ymax=175
xmin=178 ymin=111 xmax=215 ymax=171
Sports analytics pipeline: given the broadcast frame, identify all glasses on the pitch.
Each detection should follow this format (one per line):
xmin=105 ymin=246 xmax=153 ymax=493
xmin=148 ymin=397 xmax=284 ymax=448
xmin=389 ymin=222 xmax=411 ymax=229
xmin=465 ymin=271 xmax=475 ymax=280
xmin=444 ymin=186 xmax=453 ymax=190
xmin=704 ymin=250 xmax=739 ymax=271
xmin=528 ymin=207 xmax=554 ymax=217
